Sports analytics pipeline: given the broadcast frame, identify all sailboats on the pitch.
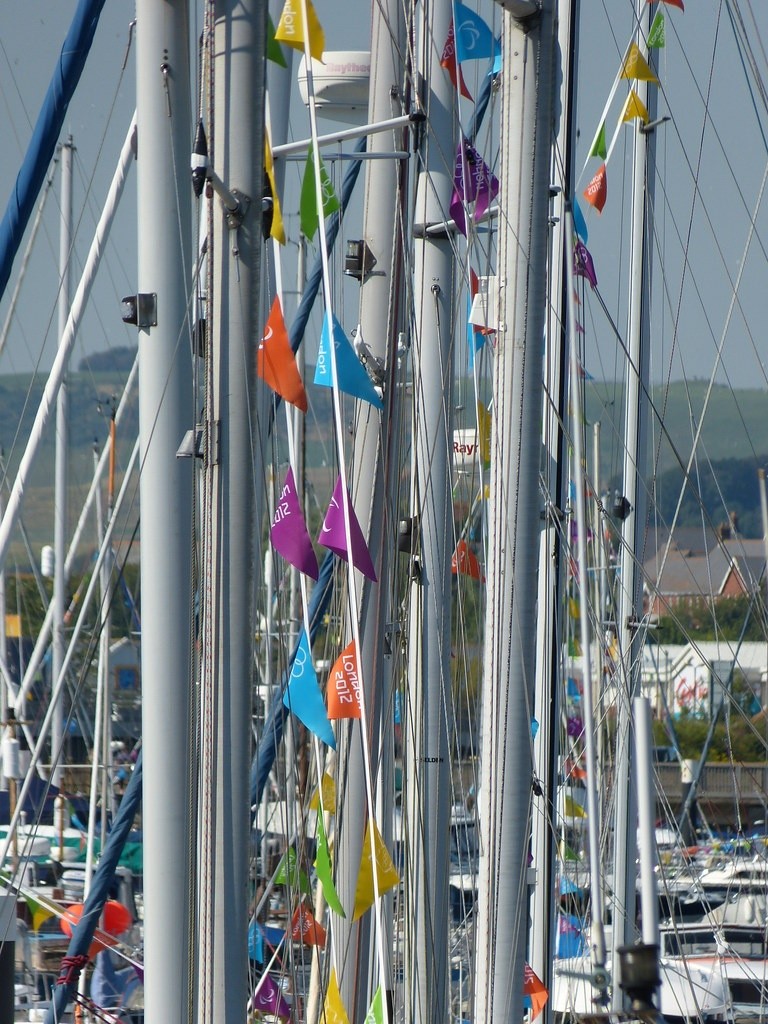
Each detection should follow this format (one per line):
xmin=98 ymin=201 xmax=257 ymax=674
xmin=1 ymin=2 xmax=766 ymax=1024
